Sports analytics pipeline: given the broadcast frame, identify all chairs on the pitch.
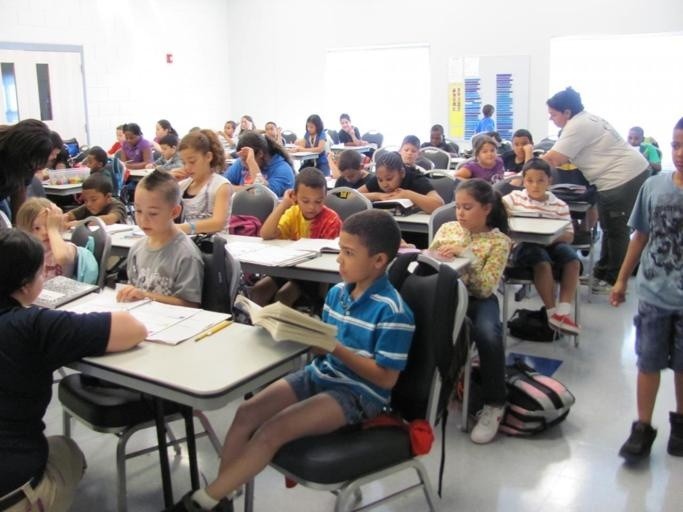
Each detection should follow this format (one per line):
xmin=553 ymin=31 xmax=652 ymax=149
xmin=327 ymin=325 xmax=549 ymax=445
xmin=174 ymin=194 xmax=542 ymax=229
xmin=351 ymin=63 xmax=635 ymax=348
xmin=412 ymin=143 xmax=454 ymax=171
xmin=228 ymin=182 xmax=280 ymax=239
xmin=240 ymin=251 xmax=471 ymax=509
xmin=54 ymin=239 xmax=240 ymax=509
xmin=59 ymin=213 xmax=114 ymax=286
xmin=409 ymin=167 xmax=460 ymax=208
xmin=314 ymin=183 xmax=380 ymax=243
xmin=424 ymin=197 xmax=495 ymax=251
xmin=499 ymin=174 xmax=584 ymax=351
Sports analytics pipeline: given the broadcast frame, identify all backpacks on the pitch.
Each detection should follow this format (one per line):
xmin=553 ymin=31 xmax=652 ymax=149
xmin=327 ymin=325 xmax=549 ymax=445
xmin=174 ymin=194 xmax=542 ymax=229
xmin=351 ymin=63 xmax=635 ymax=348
xmin=457 ymin=355 xmax=575 ymax=437
xmin=229 ymin=215 xmax=261 ymax=285
xmin=74 ymin=236 xmax=99 ymax=283
xmin=389 ymin=253 xmax=475 ymax=495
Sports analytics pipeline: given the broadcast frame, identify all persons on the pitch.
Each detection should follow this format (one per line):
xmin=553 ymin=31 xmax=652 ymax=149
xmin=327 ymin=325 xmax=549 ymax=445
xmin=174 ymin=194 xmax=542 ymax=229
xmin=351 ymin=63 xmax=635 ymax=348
xmin=540 ymin=88 xmax=652 ymax=293
xmin=428 ymin=178 xmax=512 ymax=444
xmin=250 ymin=167 xmax=342 ymax=307
xmin=501 ymin=157 xmax=581 ymax=336
xmin=1 ymin=104 xmax=662 ymax=284
xmin=172 ymin=209 xmax=415 ymax=512
xmin=116 ymin=169 xmax=204 ymax=308
xmin=609 ymin=117 xmax=683 ymax=459
xmin=1 ymin=227 xmax=147 ymax=512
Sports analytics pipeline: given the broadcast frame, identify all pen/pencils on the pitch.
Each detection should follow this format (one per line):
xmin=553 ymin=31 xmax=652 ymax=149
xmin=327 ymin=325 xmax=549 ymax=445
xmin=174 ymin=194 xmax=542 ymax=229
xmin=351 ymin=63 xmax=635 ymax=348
xmin=195 ymin=321 xmax=232 ymax=342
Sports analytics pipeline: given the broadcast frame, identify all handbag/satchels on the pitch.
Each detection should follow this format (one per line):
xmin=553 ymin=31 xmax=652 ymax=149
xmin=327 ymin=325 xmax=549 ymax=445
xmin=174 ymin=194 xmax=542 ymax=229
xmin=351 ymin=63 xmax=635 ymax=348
xmin=507 ymin=307 xmax=558 ymax=341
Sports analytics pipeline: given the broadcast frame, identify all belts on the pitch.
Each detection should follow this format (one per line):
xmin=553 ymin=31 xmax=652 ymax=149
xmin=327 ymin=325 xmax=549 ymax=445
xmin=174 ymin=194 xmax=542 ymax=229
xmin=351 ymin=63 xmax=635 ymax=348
xmin=0 ymin=474 xmax=39 ymax=511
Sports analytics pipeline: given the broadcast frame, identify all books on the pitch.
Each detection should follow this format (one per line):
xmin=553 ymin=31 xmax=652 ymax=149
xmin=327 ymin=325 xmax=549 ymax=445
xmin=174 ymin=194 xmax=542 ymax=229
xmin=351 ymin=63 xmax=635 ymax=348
xmin=31 ymin=276 xmax=99 ymax=310
xmin=231 ymin=294 xmax=339 ymax=352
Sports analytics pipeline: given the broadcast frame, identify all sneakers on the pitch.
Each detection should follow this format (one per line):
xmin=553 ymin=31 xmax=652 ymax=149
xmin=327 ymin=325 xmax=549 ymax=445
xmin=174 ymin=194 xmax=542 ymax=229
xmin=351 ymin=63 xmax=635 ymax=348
xmin=548 ymin=311 xmax=580 ymax=336
xmin=470 ymin=405 xmax=505 ymax=443
xmin=620 ymin=421 xmax=656 ymax=459
xmin=668 ymin=412 xmax=683 ymax=456
